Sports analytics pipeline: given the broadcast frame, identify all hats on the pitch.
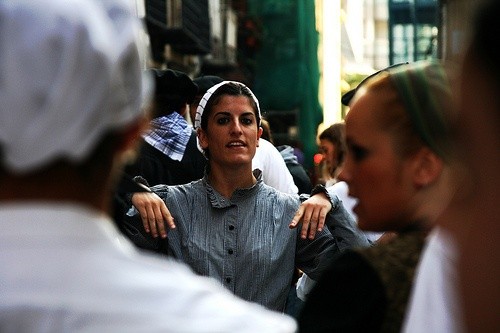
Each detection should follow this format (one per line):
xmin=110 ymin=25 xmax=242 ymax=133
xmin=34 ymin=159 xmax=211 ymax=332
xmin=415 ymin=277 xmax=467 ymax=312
xmin=0 ymin=0 xmax=156 ymax=172
xmin=152 ymin=69 xmax=197 ymax=105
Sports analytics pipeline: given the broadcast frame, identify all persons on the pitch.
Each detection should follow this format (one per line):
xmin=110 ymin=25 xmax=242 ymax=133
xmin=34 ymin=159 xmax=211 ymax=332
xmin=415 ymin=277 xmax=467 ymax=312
xmin=0 ymin=0 xmax=500 ymax=333
xmin=127 ymin=80 xmax=372 ymax=315
